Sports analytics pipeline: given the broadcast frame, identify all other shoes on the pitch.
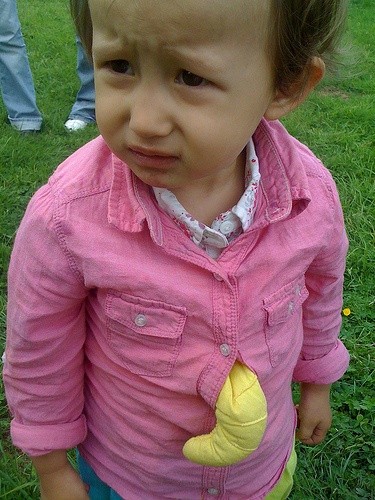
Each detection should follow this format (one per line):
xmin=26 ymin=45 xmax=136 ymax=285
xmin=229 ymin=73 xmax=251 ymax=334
xmin=65 ymin=119 xmax=87 ymax=132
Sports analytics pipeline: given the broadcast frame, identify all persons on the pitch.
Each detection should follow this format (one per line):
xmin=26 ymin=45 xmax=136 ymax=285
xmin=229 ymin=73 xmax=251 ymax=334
xmin=0 ymin=0 xmax=43 ymax=132
xmin=2 ymin=0 xmax=350 ymax=500
xmin=64 ymin=36 xmax=96 ymax=131
